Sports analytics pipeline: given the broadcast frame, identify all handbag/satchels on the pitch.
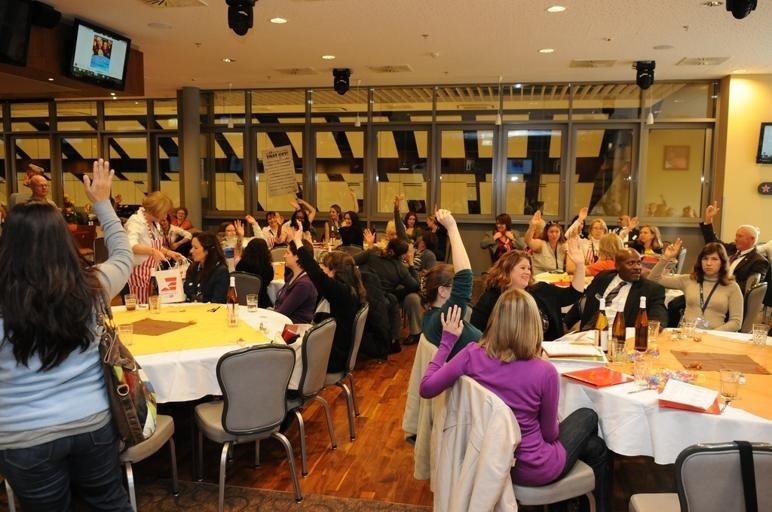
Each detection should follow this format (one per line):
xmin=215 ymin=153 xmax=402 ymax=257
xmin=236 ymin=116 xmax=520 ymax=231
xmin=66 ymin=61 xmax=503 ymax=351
xmin=153 ymin=259 xmax=189 ymax=305
xmin=89 ymin=268 xmax=158 ymax=447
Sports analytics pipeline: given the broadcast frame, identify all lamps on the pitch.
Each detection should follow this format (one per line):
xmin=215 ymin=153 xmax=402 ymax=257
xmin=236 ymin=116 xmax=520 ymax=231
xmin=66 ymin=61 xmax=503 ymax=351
xmin=634 ymin=61 xmax=656 ymax=91
xmin=224 ymin=0 xmax=257 ymax=36
xmin=333 ymin=67 xmax=351 ymax=96
xmin=647 ymin=85 xmax=654 ymax=126
xmin=354 ymin=78 xmax=364 ymax=129
xmin=226 ymin=83 xmax=234 ymax=131
xmin=494 ymin=76 xmax=503 ymax=128
xmin=724 ymin=1 xmax=760 ymax=21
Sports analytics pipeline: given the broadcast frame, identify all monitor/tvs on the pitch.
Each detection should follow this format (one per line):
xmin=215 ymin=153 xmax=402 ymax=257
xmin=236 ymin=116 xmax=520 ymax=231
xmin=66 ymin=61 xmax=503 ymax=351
xmin=65 ymin=17 xmax=131 ymax=91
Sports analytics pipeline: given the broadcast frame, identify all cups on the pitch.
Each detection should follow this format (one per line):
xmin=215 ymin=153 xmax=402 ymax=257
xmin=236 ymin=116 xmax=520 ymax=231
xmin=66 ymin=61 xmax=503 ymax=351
xmin=647 ymin=318 xmax=661 ymax=341
xmin=246 ymin=293 xmax=258 ymax=312
xmin=124 ymin=294 xmax=138 ymax=311
xmin=612 ymin=341 xmax=627 ymax=364
xmin=719 ymin=369 xmax=739 ymax=399
xmin=117 ymin=324 xmax=134 ymax=346
xmin=150 ymin=295 xmax=161 ymax=313
xmin=678 ymin=320 xmax=693 ymax=340
xmin=751 ymin=324 xmax=769 ymax=347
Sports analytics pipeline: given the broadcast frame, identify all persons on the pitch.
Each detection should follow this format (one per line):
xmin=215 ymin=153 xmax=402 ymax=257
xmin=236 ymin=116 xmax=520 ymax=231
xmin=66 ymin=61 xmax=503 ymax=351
xmin=111 ymin=192 xmax=482 ymax=373
xmin=469 ymin=201 xmax=772 ymax=342
xmin=419 ymin=287 xmax=612 ymax=509
xmin=5 ymin=174 xmax=97 ymax=225
xmin=0 ymin=158 xmax=136 ymax=512
xmin=94 ymin=35 xmax=114 ymax=59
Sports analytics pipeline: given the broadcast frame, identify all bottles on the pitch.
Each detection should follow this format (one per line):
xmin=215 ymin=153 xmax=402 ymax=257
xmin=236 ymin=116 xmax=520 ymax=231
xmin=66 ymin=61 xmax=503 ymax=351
xmin=634 ymin=356 xmax=650 ymax=385
xmin=227 ymin=276 xmax=239 ymax=325
xmin=371 ymin=223 xmax=377 ymax=243
xmin=329 ymin=225 xmax=341 ymax=246
xmin=148 ymin=267 xmax=159 ymax=310
xmin=611 ymin=300 xmax=626 ymax=355
xmin=691 ymin=316 xmax=704 ymax=341
xmin=634 ymin=296 xmax=648 ymax=351
xmin=595 ymin=297 xmax=608 ymax=353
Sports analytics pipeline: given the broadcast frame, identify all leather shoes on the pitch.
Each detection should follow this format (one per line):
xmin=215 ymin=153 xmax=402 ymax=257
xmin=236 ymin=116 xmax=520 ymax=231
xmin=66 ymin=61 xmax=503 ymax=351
xmin=401 ymin=335 xmax=420 ymax=346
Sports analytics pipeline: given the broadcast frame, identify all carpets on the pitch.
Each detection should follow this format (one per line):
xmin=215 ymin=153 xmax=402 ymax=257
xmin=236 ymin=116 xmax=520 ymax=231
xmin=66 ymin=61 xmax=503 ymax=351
xmin=126 ymin=478 xmax=437 ymax=510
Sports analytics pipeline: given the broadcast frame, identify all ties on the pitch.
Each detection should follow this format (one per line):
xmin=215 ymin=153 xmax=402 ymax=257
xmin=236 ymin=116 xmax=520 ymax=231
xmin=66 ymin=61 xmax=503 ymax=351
xmin=615 ymin=227 xmax=622 ymax=236
xmin=728 ymin=250 xmax=741 ymax=267
xmin=605 ymin=281 xmax=629 ymax=307
